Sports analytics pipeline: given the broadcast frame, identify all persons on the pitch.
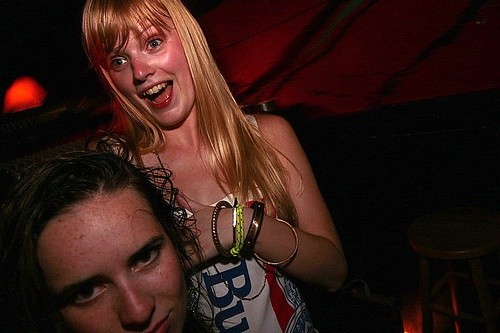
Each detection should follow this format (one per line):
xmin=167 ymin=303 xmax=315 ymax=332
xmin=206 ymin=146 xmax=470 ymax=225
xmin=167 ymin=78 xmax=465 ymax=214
xmin=0 ymin=134 xmax=217 ymax=333
xmin=79 ymin=0 xmax=351 ymax=332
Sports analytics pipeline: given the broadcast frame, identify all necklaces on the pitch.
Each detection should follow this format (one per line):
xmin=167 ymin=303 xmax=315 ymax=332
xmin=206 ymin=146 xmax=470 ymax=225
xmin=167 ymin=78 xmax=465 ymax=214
xmin=149 ymin=136 xmax=183 ymax=207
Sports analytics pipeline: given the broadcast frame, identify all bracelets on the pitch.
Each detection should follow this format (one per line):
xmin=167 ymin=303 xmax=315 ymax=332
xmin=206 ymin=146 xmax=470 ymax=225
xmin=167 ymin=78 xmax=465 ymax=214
xmin=237 ymin=200 xmax=266 ymax=260
xmin=230 ymin=202 xmax=245 ymax=257
xmin=253 ymin=216 xmax=300 ymax=266
xmin=211 ymin=199 xmax=231 ymax=258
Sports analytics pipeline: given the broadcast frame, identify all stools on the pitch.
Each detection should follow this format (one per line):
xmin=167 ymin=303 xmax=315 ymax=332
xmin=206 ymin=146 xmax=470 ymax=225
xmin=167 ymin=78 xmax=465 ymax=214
xmin=407 ymin=207 xmax=500 ymax=333
